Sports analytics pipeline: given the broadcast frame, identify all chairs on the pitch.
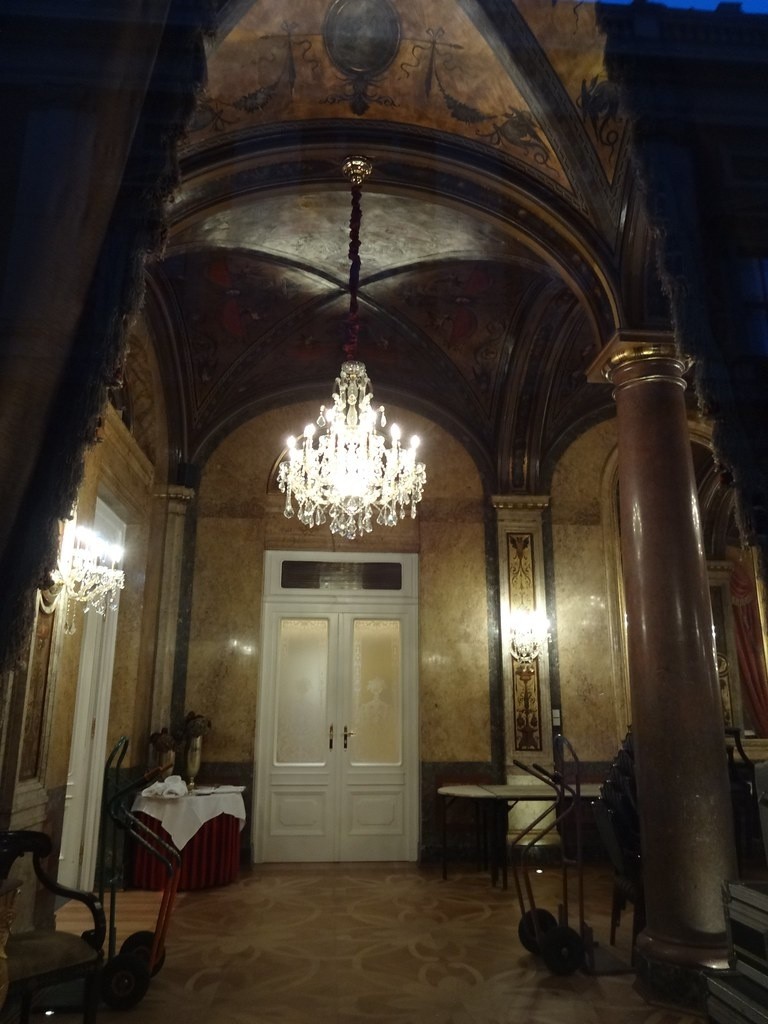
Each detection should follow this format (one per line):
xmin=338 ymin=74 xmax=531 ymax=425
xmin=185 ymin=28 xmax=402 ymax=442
xmin=0 ymin=829 xmax=108 ymax=1024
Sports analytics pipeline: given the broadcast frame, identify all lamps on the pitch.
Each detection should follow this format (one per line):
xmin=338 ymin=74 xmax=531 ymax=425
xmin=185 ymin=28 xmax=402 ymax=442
xmin=504 ymin=607 xmax=554 ymax=674
xmin=46 ymin=491 xmax=126 ymax=636
xmin=271 ymin=152 xmax=427 ymax=540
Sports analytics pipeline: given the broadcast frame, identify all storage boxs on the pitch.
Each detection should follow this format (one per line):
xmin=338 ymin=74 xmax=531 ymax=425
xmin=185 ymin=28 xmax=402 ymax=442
xmin=698 ymin=967 xmax=768 ymax=1024
xmin=719 ymin=876 xmax=768 ymax=991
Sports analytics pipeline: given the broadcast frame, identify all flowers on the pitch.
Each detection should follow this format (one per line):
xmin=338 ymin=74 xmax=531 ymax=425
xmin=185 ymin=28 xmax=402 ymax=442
xmin=184 ymin=710 xmax=211 ymax=737
xmin=148 ymin=726 xmax=188 ymax=754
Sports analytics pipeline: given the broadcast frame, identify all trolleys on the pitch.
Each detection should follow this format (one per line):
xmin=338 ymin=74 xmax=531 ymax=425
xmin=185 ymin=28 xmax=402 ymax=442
xmin=79 ymin=734 xmax=185 ymax=1008
xmin=508 ymin=732 xmax=639 ymax=982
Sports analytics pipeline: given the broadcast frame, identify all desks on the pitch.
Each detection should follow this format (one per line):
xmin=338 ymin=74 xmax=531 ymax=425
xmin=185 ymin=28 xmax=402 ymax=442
xmin=123 ymin=785 xmax=248 ymax=892
xmin=436 ymin=781 xmax=603 ymax=891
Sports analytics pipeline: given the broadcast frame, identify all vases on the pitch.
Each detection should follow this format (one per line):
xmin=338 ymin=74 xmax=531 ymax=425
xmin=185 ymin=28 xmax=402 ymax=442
xmin=182 ymin=731 xmax=202 ymax=787
xmin=160 ymin=750 xmax=175 ymax=781
